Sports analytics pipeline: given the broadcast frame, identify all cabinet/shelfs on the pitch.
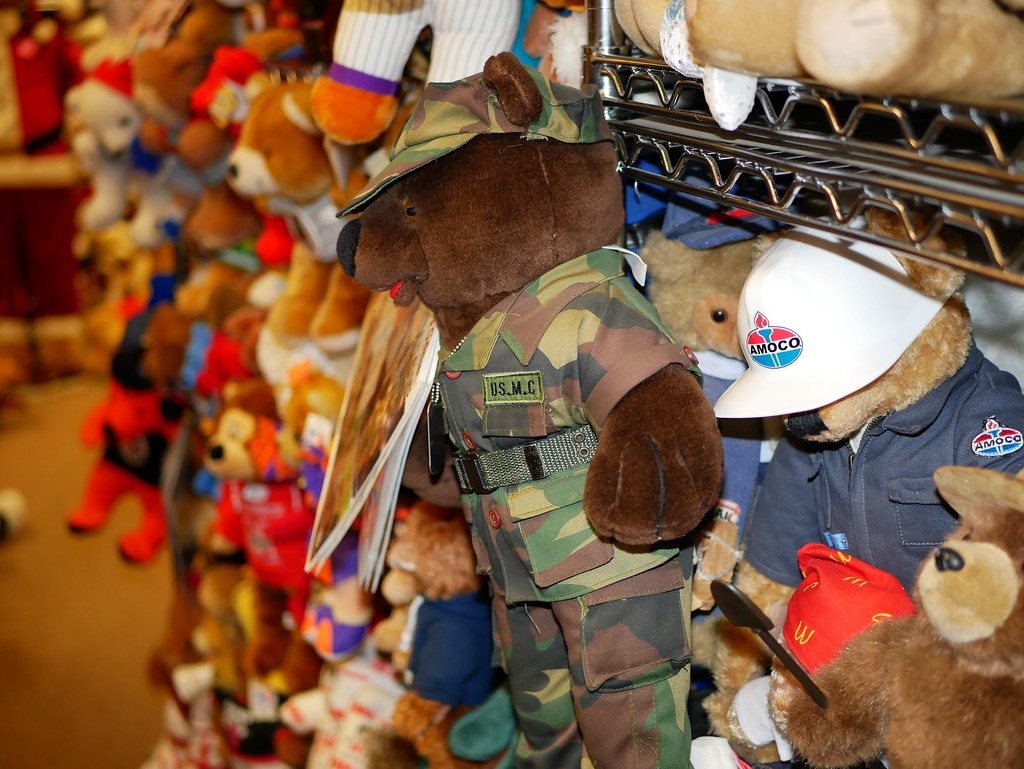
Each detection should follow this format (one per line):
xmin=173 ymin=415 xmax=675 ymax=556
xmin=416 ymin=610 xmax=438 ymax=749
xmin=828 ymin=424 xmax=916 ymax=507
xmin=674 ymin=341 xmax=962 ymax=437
xmin=579 ymin=0 xmax=1024 ymax=295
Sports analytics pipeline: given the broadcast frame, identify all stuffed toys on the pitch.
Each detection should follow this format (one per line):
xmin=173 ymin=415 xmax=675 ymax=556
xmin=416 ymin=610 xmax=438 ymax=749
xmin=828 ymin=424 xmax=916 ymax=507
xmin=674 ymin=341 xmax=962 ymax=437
xmin=0 ymin=0 xmax=1024 ymax=769
